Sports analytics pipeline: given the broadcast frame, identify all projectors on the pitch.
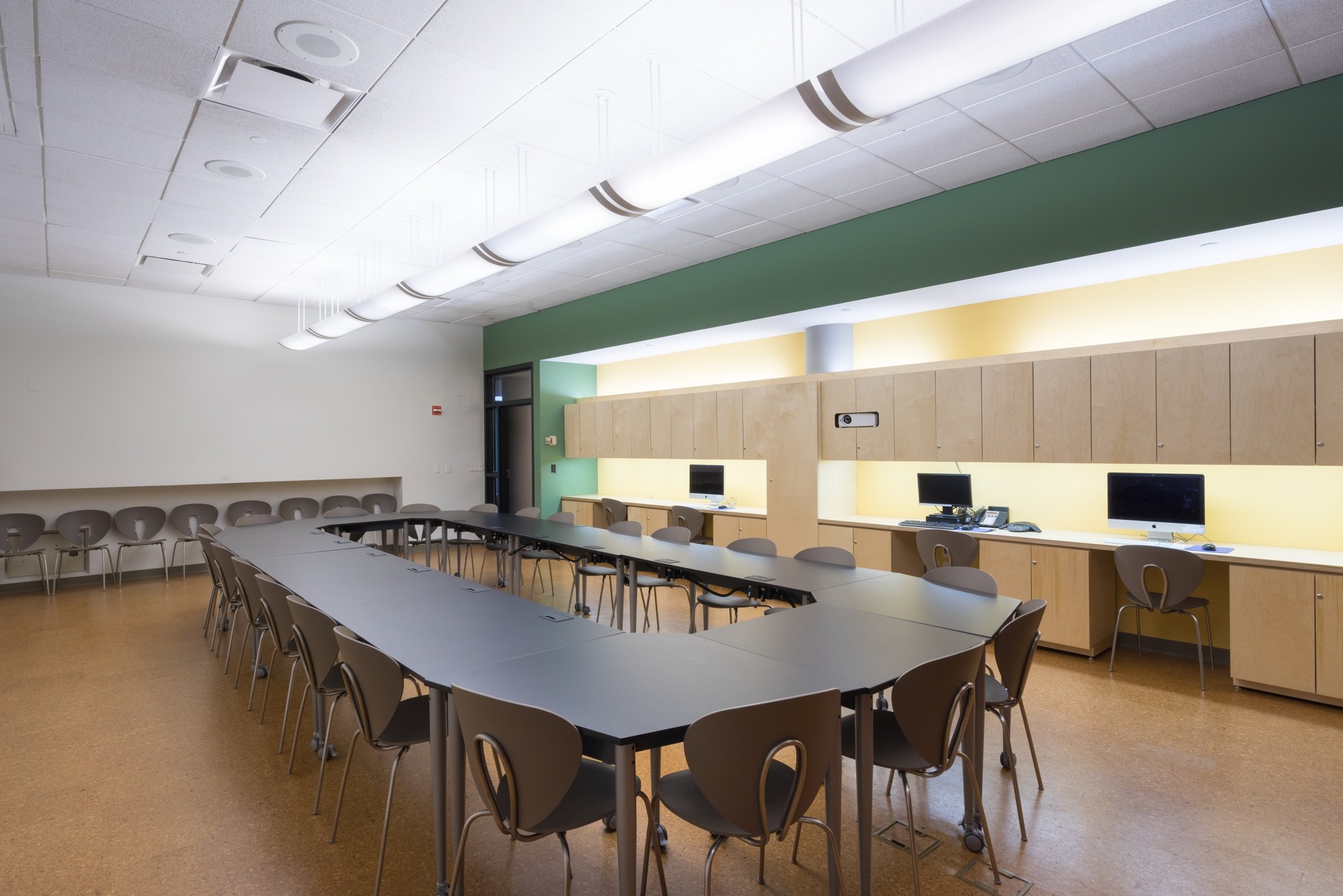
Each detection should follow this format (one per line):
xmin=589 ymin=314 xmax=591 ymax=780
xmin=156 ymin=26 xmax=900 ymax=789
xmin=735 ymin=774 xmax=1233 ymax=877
xmin=835 ymin=410 xmax=882 ymax=427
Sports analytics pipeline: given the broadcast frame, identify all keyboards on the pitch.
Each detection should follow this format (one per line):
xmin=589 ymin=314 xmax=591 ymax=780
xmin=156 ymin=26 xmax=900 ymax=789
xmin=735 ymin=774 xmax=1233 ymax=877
xmin=686 ymin=504 xmax=711 ymax=509
xmin=898 ymin=519 xmax=960 ymax=529
xmin=1105 ymin=538 xmax=1187 ymax=550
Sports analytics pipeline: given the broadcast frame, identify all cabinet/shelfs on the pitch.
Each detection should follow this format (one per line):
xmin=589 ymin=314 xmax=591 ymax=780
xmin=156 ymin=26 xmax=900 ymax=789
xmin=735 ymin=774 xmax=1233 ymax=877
xmin=564 ymin=402 xmax=597 ymax=458
xmin=764 ymin=380 xmax=818 ymax=558
xmin=716 ymin=385 xmax=766 ymax=460
xmin=979 ymin=538 xmax=1117 ymax=650
xmin=630 ymin=394 xmax=673 ymax=459
xmin=893 ymin=365 xmax=983 ymax=462
xmin=1230 ymin=331 xmax=1343 ymax=466
xmin=981 ymin=355 xmax=1092 ymax=464
xmin=821 ymin=374 xmax=895 ymax=461
xmin=1229 ymin=562 xmax=1343 ymax=700
xmin=595 ymin=399 xmax=632 ymax=458
xmin=1090 ymin=342 xmax=1232 ymax=465
xmin=669 ymin=391 xmax=718 ymax=460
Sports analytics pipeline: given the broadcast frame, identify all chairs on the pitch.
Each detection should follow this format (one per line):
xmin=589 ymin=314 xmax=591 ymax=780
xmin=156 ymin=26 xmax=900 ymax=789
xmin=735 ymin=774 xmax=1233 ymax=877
xmin=639 ymin=686 xmax=849 ymax=896
xmin=169 ymin=493 xmax=859 ymax=777
xmin=448 ymin=683 xmax=670 ymax=896
xmin=114 ymin=506 xmax=169 ymax=588
xmin=52 ymin=510 xmax=116 ymax=596
xmin=0 ymin=513 xmax=50 ymax=597
xmin=885 ymin=599 xmax=1049 ymax=841
xmin=286 ymin=594 xmax=423 ymax=815
xmin=839 ymin=639 xmax=1002 ymax=896
xmin=1107 ymin=544 xmax=1216 ymax=691
xmin=327 ymin=626 xmax=450 ymax=896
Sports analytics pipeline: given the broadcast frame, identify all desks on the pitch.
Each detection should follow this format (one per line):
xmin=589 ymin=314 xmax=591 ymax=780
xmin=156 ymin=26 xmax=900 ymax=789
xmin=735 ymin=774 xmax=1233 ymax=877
xmin=215 ymin=510 xmax=1022 ymax=896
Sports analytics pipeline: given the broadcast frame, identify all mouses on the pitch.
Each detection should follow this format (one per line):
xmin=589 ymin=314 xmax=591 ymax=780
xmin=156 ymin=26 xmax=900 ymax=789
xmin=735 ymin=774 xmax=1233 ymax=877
xmin=1202 ymin=543 xmax=1216 ymax=551
xmin=963 ymin=524 xmax=973 ymax=530
xmin=718 ymin=505 xmax=727 ymax=509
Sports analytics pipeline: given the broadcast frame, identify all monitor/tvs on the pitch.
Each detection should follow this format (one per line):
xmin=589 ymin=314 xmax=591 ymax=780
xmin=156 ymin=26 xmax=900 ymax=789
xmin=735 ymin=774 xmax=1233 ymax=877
xmin=688 ymin=464 xmax=724 ymax=502
xmin=1107 ymin=472 xmax=1206 ymax=534
xmin=917 ymin=473 xmax=971 ymax=506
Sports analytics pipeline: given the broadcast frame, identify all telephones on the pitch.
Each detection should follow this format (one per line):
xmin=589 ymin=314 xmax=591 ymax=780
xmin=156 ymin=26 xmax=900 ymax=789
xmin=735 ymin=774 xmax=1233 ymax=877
xmin=998 ymin=521 xmax=1042 ymax=532
xmin=971 ymin=508 xmax=1008 ymax=528
